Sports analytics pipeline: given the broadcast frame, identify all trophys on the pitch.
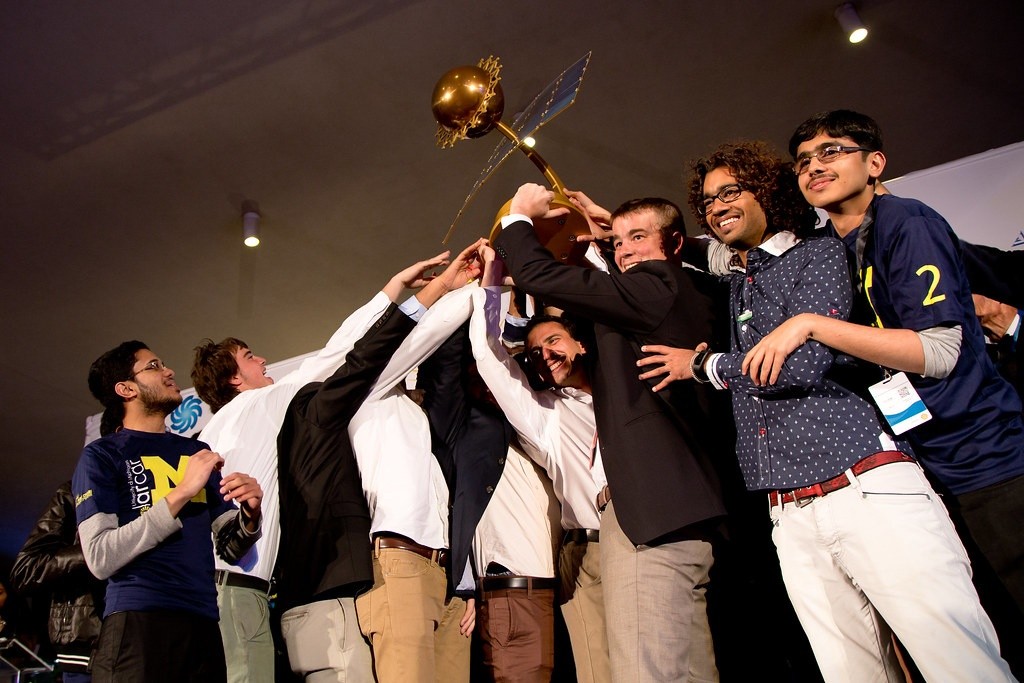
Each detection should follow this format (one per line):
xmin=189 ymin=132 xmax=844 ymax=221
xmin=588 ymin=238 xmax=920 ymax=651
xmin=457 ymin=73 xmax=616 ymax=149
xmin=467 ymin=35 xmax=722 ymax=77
xmin=430 ymin=49 xmax=593 ymax=263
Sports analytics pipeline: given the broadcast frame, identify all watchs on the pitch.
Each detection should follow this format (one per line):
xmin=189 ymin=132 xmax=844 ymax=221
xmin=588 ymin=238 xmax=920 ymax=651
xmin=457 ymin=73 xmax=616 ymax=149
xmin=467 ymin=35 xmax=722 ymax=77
xmin=689 ymin=350 xmax=710 ymax=383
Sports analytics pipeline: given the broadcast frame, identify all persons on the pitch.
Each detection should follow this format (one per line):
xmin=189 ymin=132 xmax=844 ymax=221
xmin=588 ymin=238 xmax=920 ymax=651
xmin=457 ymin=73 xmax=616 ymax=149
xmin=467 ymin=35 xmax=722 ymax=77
xmin=0 ymin=575 xmax=7 ymax=642
xmin=11 ymin=410 xmax=124 ymax=683
xmin=70 ymin=340 xmax=263 ymax=683
xmin=191 ymin=107 xmax=1024 ymax=683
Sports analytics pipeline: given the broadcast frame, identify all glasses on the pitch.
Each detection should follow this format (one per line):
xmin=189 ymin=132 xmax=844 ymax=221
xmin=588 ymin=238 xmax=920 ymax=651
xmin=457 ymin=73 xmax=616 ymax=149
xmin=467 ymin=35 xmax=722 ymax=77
xmin=792 ymin=145 xmax=876 ymax=176
xmin=695 ymin=181 xmax=757 ymax=215
xmin=126 ymin=360 xmax=170 ymax=380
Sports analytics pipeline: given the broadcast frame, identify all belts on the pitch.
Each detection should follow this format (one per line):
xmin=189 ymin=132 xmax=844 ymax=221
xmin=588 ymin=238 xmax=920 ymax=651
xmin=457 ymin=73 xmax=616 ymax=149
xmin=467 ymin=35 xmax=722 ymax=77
xmin=370 ymin=537 xmax=449 ymax=569
xmin=214 ymin=569 xmax=277 ymax=596
xmin=562 ymin=528 xmax=599 ymax=545
xmin=483 ymin=577 xmax=556 ymax=592
xmin=595 ymin=485 xmax=612 ymax=513
xmin=769 ymin=450 xmax=916 ymax=508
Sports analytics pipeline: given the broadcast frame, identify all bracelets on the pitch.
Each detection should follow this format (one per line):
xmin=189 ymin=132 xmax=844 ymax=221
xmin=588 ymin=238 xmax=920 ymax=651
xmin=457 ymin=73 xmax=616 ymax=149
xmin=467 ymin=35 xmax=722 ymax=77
xmin=689 ymin=352 xmax=703 ymax=383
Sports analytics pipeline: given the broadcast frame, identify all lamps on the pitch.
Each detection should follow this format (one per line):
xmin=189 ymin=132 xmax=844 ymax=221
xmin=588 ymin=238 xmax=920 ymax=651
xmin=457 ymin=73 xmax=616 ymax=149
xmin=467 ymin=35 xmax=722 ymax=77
xmin=241 ymin=210 xmax=261 ymax=248
xmin=510 ymin=111 xmax=536 ymax=149
xmin=833 ymin=2 xmax=869 ymax=46
